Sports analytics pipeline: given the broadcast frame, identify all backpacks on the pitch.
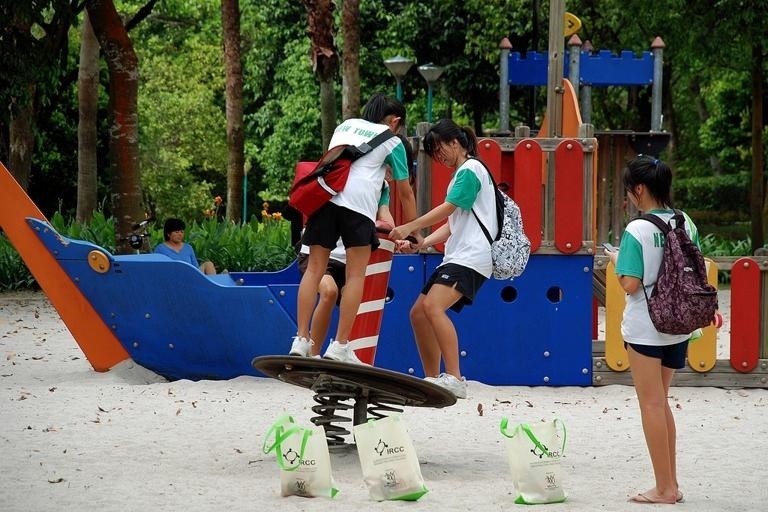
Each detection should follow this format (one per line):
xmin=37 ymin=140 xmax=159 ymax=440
xmin=629 ymin=209 xmax=718 ymax=334
xmin=458 ymin=157 xmax=531 ymax=280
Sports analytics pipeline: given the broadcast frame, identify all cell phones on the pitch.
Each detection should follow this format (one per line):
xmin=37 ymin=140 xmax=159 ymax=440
xmin=602 ymin=242 xmax=616 ymax=252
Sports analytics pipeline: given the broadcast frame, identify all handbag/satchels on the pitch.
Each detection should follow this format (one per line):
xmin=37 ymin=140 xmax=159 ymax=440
xmin=263 ymin=415 xmax=338 ymax=498
xmin=289 ymin=145 xmax=363 ymax=217
xmin=499 ymin=415 xmax=567 ymax=504
xmin=352 ymin=413 xmax=428 ymax=501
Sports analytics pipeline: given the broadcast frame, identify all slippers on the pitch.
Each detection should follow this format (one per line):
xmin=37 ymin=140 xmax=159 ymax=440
xmin=631 ymin=490 xmax=683 ymax=503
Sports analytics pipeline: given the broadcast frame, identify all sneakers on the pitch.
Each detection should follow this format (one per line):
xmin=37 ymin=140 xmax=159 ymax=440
xmin=322 ymin=337 xmax=374 ymax=366
xmin=423 ymin=372 xmax=468 ymax=399
xmin=289 ymin=335 xmax=315 ymax=358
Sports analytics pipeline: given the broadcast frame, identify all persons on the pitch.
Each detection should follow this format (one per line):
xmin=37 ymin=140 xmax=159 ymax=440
xmin=386 ymin=117 xmax=502 ymax=399
xmin=296 ymin=162 xmax=398 ymax=359
xmin=287 ymin=91 xmax=424 ymax=366
xmin=604 ymin=153 xmax=718 ymax=505
xmin=155 ymin=218 xmax=217 ymax=274
